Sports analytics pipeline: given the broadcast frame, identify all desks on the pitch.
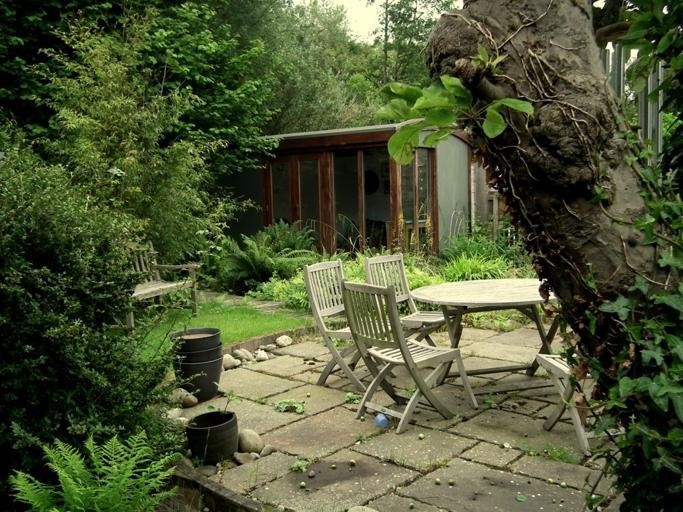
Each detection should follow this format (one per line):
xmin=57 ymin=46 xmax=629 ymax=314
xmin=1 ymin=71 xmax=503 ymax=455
xmin=410 ymin=277 xmax=563 ymax=410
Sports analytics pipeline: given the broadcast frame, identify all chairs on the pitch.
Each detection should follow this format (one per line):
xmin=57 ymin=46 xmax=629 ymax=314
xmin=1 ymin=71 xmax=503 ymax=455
xmin=122 ymin=242 xmax=201 ymax=329
xmin=536 ymin=353 xmax=613 ymax=458
xmin=303 ymin=252 xmax=479 ymax=433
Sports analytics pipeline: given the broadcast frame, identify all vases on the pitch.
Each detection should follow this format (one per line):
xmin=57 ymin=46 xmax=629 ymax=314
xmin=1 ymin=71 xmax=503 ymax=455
xmin=167 ymin=328 xmax=223 ymax=401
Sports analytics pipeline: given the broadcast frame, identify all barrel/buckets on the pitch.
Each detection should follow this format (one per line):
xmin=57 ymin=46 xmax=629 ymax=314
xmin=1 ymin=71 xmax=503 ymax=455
xmin=169 ymin=326 xmax=223 ymax=403
